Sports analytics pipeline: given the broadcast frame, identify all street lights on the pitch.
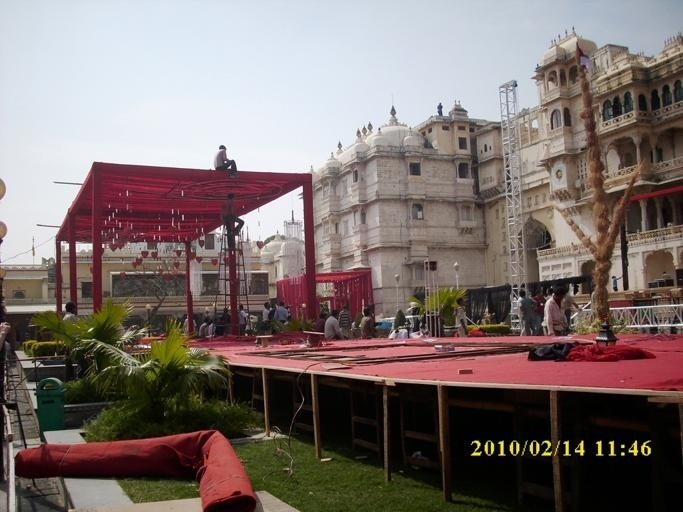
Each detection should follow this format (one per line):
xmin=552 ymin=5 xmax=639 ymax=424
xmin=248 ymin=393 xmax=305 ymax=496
xmin=395 ymin=274 xmax=399 ymax=310
xmin=453 ymin=261 xmax=459 ymax=289
xmin=0 ymin=179 xmax=8 ymax=483
xmin=146 ymin=304 xmax=152 ymax=337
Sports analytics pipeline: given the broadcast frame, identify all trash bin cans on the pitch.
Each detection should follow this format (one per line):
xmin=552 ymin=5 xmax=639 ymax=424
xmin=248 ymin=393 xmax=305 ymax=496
xmin=35 ymin=378 xmax=67 ymax=442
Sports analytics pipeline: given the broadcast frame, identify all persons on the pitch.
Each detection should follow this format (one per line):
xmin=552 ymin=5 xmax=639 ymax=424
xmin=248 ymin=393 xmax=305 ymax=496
xmin=318 ymin=303 xmax=377 ymax=340
xmin=183 ymin=304 xmax=247 ymax=337
xmin=455 ymin=297 xmax=468 ymax=337
xmin=611 ymin=274 xmax=623 ymax=292
xmin=60 ymin=301 xmax=81 ymax=332
xmin=262 ymin=300 xmax=291 ymax=324
xmin=219 ymin=192 xmax=245 ymax=248
xmin=662 ymin=272 xmax=670 ymax=278
xmin=517 ymin=284 xmax=583 ymax=336
xmin=213 ymin=145 xmax=238 ymax=177
